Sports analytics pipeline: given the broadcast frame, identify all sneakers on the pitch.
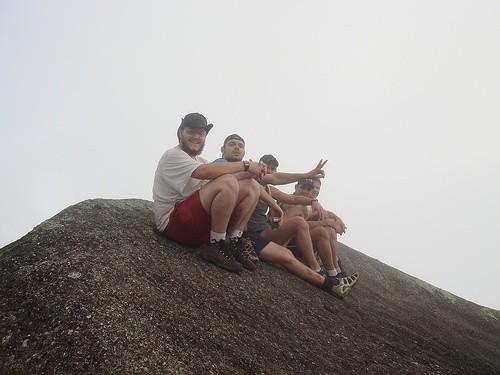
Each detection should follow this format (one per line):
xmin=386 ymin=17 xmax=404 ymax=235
xmin=317 ymin=269 xmax=329 ymax=278
xmin=238 ymin=235 xmax=259 ymax=262
xmin=228 ymin=237 xmax=256 ymax=271
xmin=202 ymin=239 xmax=243 ymax=272
xmin=329 ymin=258 xmax=349 ymax=277
xmin=330 ymin=275 xmax=359 ymax=299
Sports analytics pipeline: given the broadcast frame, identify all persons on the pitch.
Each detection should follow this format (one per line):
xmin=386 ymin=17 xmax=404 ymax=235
xmin=152 ymin=113 xmax=260 ymax=273
xmin=210 ymin=133 xmax=359 ymax=301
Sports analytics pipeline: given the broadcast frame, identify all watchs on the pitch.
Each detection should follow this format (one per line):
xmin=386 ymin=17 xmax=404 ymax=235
xmin=243 ymin=160 xmax=250 ymax=172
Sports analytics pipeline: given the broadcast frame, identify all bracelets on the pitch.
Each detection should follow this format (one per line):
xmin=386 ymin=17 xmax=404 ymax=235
xmin=310 ymin=199 xmax=318 ymax=204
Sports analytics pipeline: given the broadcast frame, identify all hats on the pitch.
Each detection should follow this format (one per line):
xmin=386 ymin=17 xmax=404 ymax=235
xmin=259 ymin=154 xmax=278 ymax=168
xmin=224 ymin=134 xmax=244 ymax=144
xmin=177 ymin=112 xmax=214 ymax=139
xmin=298 ymin=178 xmax=313 ymax=187
